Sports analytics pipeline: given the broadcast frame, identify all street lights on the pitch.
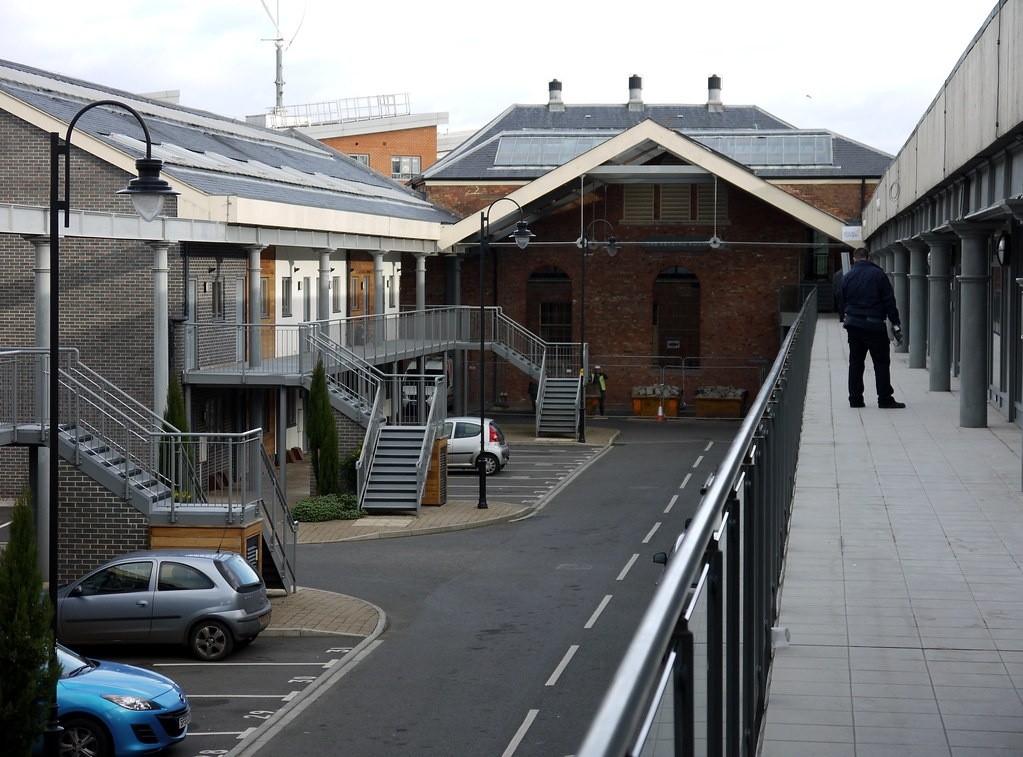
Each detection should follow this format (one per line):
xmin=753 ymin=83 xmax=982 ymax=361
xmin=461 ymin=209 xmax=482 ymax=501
xmin=45 ymin=99 xmax=182 ymax=757
xmin=478 ymin=198 xmax=537 ymax=508
xmin=578 ymin=219 xmax=622 ymax=443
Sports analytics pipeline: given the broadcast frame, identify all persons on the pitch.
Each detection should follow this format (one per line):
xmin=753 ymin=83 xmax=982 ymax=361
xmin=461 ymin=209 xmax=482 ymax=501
xmin=591 ymin=365 xmax=609 ymax=415
xmin=832 ymin=262 xmax=853 ymax=322
xmin=837 ymin=247 xmax=906 ymax=408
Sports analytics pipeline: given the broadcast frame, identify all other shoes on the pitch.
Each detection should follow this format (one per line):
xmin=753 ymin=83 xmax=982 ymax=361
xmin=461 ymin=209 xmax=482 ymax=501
xmin=850 ymin=400 xmax=865 ymax=407
xmin=878 ymin=396 xmax=905 ymax=408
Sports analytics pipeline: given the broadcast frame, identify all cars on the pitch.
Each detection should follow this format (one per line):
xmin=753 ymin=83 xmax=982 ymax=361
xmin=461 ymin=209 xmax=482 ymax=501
xmin=39 ymin=549 xmax=272 ymax=660
xmin=432 ymin=416 xmax=510 ymax=476
xmin=24 ymin=632 xmax=191 ymax=757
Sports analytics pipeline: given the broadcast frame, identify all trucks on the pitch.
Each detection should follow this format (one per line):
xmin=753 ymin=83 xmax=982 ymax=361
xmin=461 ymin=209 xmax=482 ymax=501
xmin=402 ymin=355 xmax=453 ymax=411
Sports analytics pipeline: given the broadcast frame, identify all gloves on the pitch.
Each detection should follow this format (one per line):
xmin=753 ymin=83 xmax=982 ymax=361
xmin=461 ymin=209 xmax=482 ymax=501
xmin=892 ymin=326 xmax=903 ymax=346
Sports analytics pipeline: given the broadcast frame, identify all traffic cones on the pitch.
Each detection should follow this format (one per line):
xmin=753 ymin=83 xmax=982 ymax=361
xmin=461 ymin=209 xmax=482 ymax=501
xmin=655 ymin=406 xmax=666 ymax=423
